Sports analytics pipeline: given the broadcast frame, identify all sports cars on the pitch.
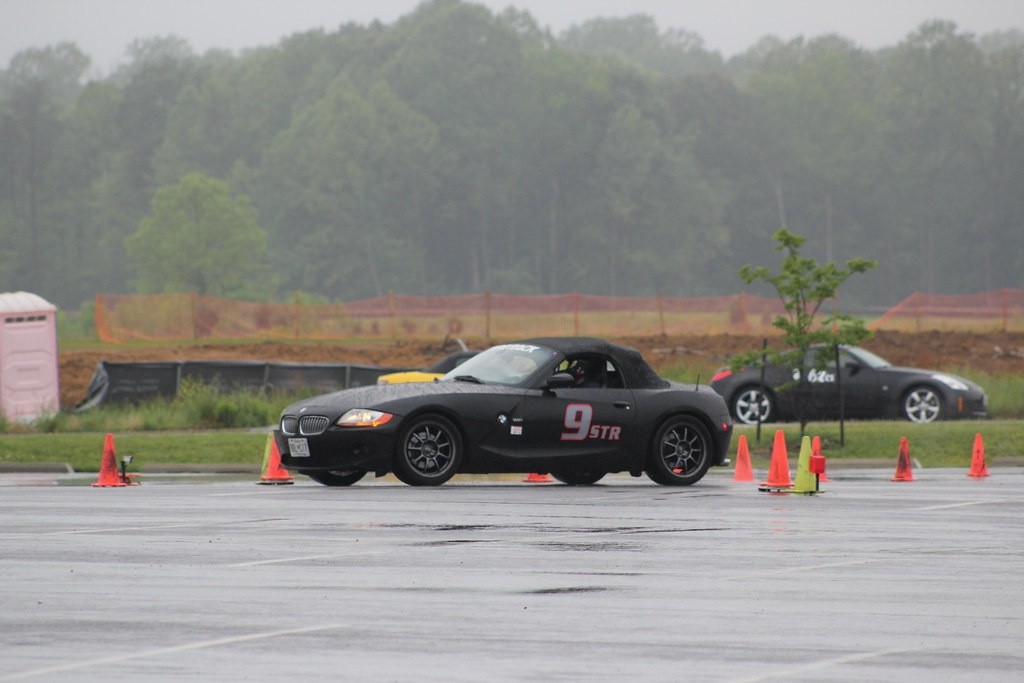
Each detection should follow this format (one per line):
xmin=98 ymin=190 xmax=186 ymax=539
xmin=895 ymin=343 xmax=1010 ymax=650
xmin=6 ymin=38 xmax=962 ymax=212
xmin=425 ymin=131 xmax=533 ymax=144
xmin=273 ymin=336 xmax=733 ymax=489
xmin=709 ymin=341 xmax=990 ymax=425
xmin=376 ymin=349 xmax=583 ymax=386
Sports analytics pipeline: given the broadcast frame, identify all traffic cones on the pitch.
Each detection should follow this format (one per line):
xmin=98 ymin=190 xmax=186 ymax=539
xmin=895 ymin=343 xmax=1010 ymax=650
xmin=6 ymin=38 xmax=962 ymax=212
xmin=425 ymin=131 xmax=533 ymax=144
xmin=89 ymin=433 xmax=127 ymax=488
xmin=965 ymin=432 xmax=991 ymax=477
xmin=787 ymin=436 xmax=824 ymax=493
xmin=522 ymin=471 xmax=553 ymax=482
xmin=758 ymin=429 xmax=794 ymax=488
xmin=256 ymin=435 xmax=296 ymax=484
xmin=811 ymin=434 xmax=831 ymax=483
xmin=890 ymin=436 xmax=916 ymax=482
xmin=732 ymin=434 xmax=756 ymax=482
